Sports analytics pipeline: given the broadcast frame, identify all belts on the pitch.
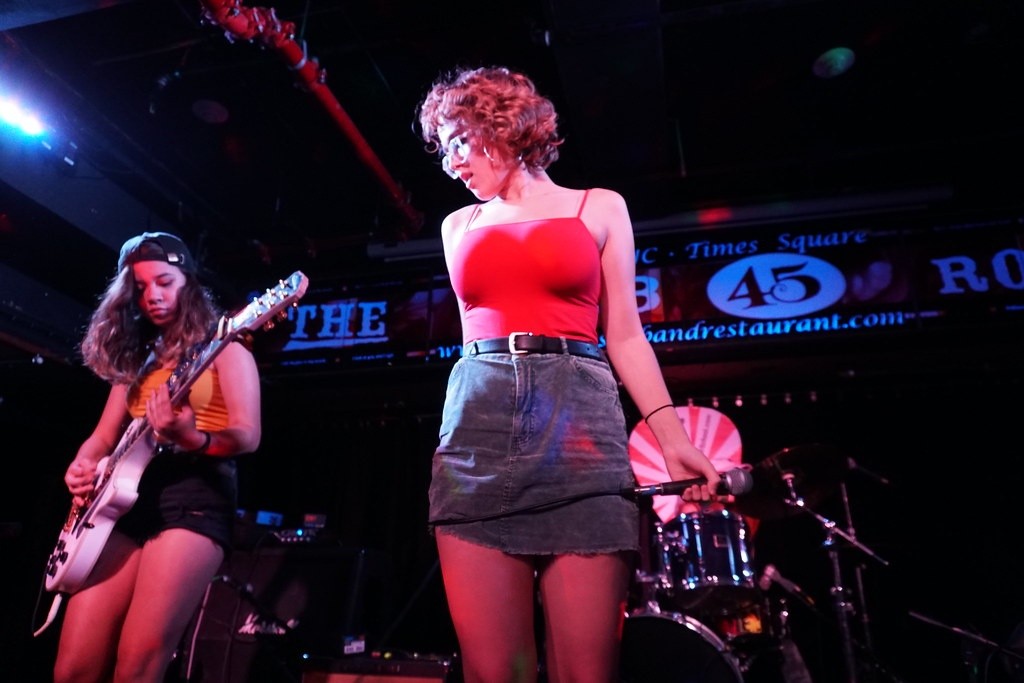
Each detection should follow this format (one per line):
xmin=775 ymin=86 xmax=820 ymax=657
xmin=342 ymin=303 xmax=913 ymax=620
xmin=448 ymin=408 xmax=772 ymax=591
xmin=463 ymin=332 xmax=609 ymax=363
xmin=152 ymin=444 xmax=184 ymax=458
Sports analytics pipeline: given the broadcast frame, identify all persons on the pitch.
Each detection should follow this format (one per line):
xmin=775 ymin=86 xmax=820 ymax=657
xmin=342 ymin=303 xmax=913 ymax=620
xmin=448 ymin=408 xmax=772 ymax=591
xmin=419 ymin=67 xmax=734 ymax=683
xmin=54 ymin=232 xmax=261 ymax=683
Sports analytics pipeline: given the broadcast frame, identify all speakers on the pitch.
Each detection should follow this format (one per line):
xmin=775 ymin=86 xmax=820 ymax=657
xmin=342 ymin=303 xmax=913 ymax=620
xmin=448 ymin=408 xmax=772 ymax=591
xmin=175 ymin=527 xmax=383 ymax=683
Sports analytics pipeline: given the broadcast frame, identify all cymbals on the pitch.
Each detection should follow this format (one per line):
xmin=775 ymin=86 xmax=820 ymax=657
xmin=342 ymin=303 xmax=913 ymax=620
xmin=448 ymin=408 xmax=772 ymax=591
xmin=746 ymin=441 xmax=819 ymax=471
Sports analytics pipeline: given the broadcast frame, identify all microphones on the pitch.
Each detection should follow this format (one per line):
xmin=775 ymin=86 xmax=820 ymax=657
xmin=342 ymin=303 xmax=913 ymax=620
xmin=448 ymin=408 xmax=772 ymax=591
xmin=220 ymin=573 xmax=253 ymax=594
xmin=617 ymin=469 xmax=754 ymax=499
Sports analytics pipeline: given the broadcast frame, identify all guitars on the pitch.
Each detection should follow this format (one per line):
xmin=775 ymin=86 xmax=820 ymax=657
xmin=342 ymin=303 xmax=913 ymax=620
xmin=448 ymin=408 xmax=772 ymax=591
xmin=45 ymin=270 xmax=309 ymax=593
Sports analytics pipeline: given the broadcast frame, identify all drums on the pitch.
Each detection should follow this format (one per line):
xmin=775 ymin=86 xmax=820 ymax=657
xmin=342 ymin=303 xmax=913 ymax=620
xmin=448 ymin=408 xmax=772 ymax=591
xmin=613 ymin=609 xmax=743 ymax=683
xmin=652 ymin=508 xmax=754 ymax=613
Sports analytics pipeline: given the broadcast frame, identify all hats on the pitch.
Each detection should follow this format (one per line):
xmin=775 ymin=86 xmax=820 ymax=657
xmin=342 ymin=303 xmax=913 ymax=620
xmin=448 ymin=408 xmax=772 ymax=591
xmin=117 ymin=231 xmax=201 ymax=275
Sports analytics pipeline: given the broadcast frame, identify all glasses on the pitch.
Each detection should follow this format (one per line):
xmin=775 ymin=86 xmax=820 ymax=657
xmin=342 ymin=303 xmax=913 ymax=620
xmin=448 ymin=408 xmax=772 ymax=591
xmin=442 ymin=134 xmax=469 ymax=179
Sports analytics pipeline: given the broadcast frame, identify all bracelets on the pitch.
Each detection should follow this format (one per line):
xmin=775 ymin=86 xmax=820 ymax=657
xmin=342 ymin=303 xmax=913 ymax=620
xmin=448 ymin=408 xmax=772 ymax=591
xmin=198 ymin=431 xmax=211 ymax=453
xmin=644 ymin=404 xmax=676 ymax=425
